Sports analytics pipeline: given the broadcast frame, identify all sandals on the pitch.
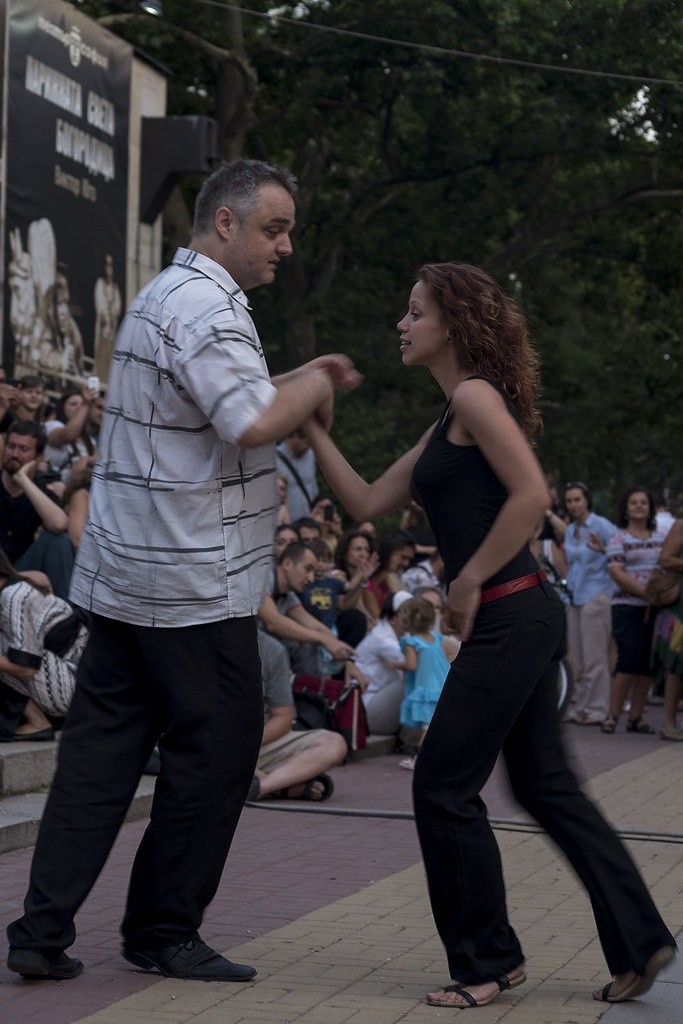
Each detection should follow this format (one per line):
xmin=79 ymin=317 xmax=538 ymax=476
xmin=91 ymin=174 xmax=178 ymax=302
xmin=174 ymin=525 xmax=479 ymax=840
xmin=599 ymin=944 xmax=675 ymax=1002
xmin=277 ymin=773 xmax=334 ymax=801
xmin=568 ymin=695 xmax=682 ymax=744
xmin=428 ymin=958 xmax=527 ymax=1006
xmin=250 ymin=776 xmax=262 ymax=799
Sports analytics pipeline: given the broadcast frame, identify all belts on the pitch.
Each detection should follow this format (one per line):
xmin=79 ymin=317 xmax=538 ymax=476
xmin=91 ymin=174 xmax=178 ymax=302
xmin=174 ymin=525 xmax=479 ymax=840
xmin=476 ymin=572 xmax=552 ymax=606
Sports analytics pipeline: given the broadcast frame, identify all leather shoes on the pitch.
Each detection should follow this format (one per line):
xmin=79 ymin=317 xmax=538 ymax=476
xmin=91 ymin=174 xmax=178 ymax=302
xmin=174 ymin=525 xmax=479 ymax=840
xmin=8 ymin=942 xmax=84 ymax=979
xmin=120 ymin=931 xmax=257 ymax=982
xmin=13 ymin=725 xmax=60 ymax=741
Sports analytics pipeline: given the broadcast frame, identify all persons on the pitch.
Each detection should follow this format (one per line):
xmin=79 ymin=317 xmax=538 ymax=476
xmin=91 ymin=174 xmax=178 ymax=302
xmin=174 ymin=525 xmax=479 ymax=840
xmin=1 ymin=157 xmax=683 ymax=1009
